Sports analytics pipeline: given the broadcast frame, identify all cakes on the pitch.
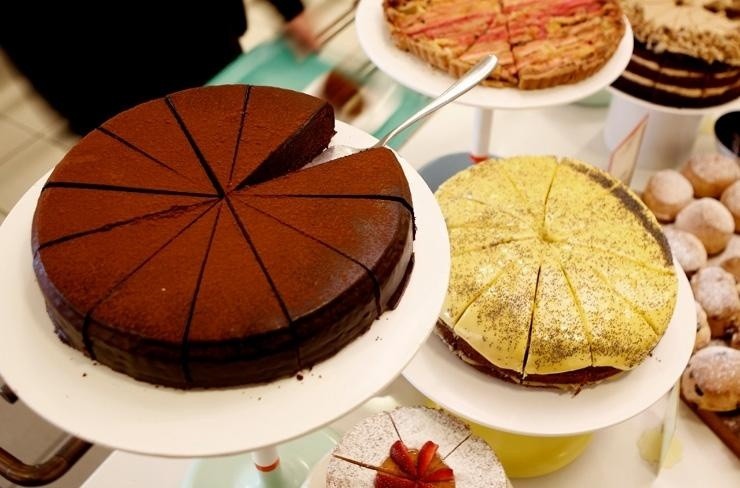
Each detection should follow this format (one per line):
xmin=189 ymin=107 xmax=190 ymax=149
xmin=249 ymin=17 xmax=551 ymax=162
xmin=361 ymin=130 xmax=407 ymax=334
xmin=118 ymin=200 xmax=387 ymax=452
xmin=665 ymin=232 xmax=706 ymax=273
xmin=675 ymin=196 xmax=733 ymax=254
xmin=326 ymin=406 xmax=514 ymax=488
xmin=432 ymin=154 xmax=677 ymax=390
xmin=683 ymin=344 xmax=740 ymax=411
xmin=382 ymin=1 xmax=625 ymax=91
xmin=616 ymin=1 xmax=740 ymax=108
xmin=684 ymin=153 xmax=736 ymax=193
xmin=32 ymin=84 xmax=412 ymax=390
xmin=692 ymin=297 xmax=710 ymax=350
xmin=675 ymin=392 xmax=676 ymax=394
xmin=723 ymin=259 xmax=738 ymax=284
xmin=723 ymin=176 xmax=740 ymax=230
xmin=642 ymin=168 xmax=695 ymax=222
xmin=691 ymin=265 xmax=735 ymax=332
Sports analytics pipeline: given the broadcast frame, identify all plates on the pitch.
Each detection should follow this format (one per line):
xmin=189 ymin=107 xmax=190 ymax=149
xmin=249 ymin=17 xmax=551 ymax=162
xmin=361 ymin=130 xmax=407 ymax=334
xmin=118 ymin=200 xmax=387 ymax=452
xmin=603 ymin=80 xmax=736 ymax=116
xmin=393 ymin=253 xmax=699 ymax=438
xmin=356 ymin=2 xmax=634 ymax=109
xmin=0 ymin=117 xmax=451 ymax=459
